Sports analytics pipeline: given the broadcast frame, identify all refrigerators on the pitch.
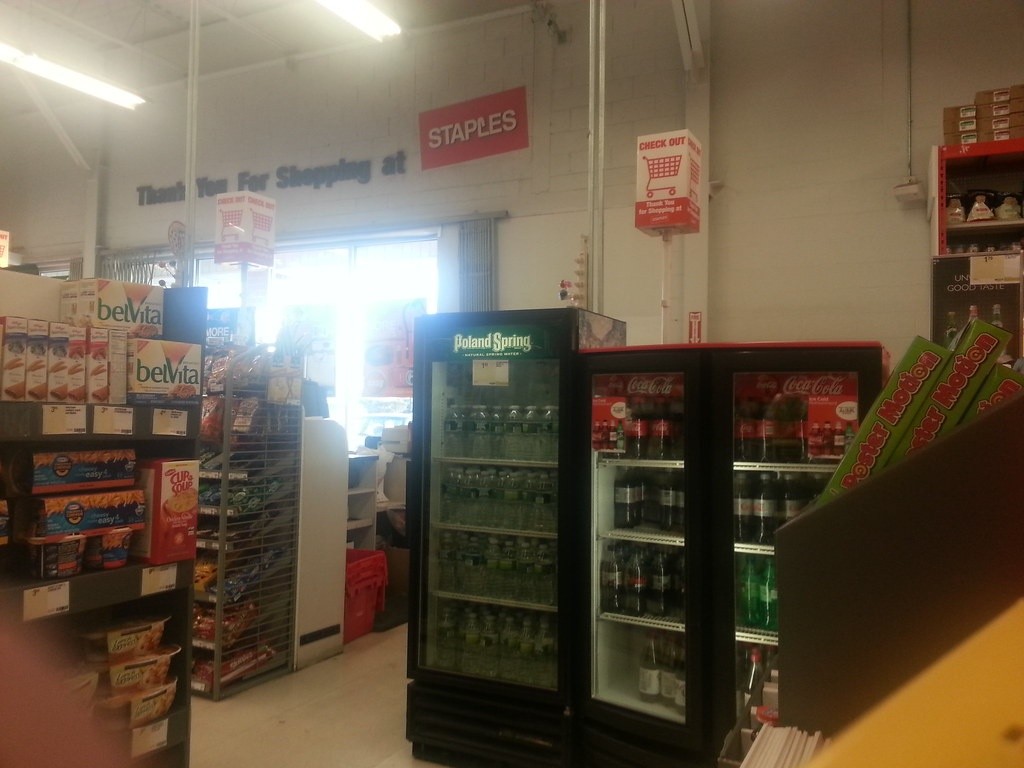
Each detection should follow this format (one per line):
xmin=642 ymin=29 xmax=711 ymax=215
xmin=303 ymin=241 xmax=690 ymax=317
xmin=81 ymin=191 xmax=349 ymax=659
xmin=406 ymin=309 xmax=626 ymax=768
xmin=579 ymin=342 xmax=888 ymax=768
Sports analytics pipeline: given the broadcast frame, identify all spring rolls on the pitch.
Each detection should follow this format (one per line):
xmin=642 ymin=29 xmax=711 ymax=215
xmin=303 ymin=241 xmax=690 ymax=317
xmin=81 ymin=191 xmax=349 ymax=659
xmin=5 ymin=382 xmax=109 ymax=402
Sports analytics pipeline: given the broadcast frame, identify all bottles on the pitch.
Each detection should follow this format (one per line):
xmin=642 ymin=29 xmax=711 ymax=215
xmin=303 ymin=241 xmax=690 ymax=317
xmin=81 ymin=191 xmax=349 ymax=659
xmin=737 ymin=644 xmax=779 ymax=725
xmin=468 ymin=405 xmax=504 ymax=459
xmin=639 ymin=630 xmax=685 ymax=714
xmin=437 ymin=603 xmax=557 ymax=688
xmin=735 ymin=396 xmax=807 ymax=463
xmin=504 ymin=405 xmax=558 ymax=462
xmin=609 ymin=421 xmax=616 ymax=448
xmin=600 ymin=541 xmax=685 ymax=617
xmin=617 ymin=421 xmax=625 ymax=449
xmin=946 ymin=305 xmax=1004 ymax=349
xmin=627 ymin=395 xmax=683 ymax=460
xmin=439 ymin=533 xmax=556 ymax=605
xmin=592 ymin=421 xmax=601 ymax=449
xmin=735 ymin=553 xmax=778 ymax=631
xmin=601 ymin=421 xmax=609 ymax=449
xmin=733 ymin=473 xmax=824 ymax=546
xmin=441 ymin=467 xmax=558 ymax=534
xmin=444 ymin=405 xmax=467 ymax=458
xmin=615 ymin=468 xmax=684 ymax=532
xmin=808 ymin=421 xmax=854 ymax=456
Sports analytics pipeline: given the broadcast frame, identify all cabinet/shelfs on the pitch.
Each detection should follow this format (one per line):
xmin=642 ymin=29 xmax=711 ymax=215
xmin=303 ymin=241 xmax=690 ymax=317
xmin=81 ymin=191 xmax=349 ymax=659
xmin=349 ymin=447 xmax=379 ymax=550
xmin=0 ymin=270 xmax=208 ymax=768
xmin=926 ymin=136 xmax=1024 ymax=256
xmin=188 ymin=342 xmax=305 ymax=703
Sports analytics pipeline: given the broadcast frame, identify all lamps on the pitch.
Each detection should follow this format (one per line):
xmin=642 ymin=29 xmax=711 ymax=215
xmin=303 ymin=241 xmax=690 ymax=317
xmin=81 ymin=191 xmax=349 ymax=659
xmin=315 ymin=0 xmax=402 ymax=42
xmin=0 ymin=41 xmax=147 ymax=110
